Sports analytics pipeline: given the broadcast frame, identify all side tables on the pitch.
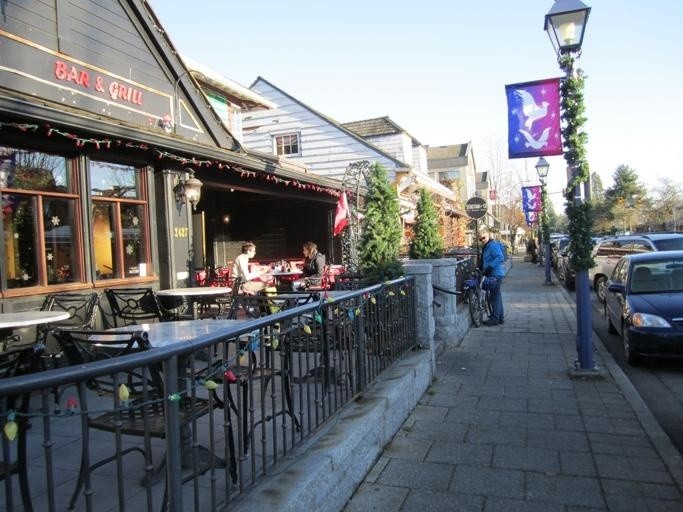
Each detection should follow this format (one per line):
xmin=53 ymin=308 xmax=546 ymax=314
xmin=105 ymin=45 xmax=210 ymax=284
xmin=91 ymin=318 xmax=248 ymax=487
xmin=1 ymin=310 xmax=70 ymax=329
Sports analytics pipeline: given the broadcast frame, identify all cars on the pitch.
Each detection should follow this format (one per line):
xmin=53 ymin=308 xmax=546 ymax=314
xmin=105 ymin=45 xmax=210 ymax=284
xmin=551 ymin=233 xmax=683 ymax=365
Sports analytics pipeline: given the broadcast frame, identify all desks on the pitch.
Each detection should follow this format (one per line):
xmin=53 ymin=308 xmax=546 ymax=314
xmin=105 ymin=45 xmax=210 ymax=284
xmin=268 ymin=289 xmax=366 ymax=403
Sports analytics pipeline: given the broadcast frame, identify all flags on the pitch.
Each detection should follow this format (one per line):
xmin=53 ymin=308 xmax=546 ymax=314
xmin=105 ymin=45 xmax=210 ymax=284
xmin=521 ymin=185 xmax=542 ymax=227
xmin=505 ymin=77 xmax=562 ymax=159
xmin=331 ymin=191 xmax=349 ymax=237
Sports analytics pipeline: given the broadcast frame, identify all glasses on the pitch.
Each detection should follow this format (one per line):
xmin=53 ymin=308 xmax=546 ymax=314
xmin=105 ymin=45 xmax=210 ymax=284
xmin=478 ymin=235 xmax=487 ymax=240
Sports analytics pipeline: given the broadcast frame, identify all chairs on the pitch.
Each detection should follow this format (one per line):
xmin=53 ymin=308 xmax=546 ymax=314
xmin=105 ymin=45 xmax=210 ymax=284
xmin=632 ymin=266 xmax=683 ymax=292
xmin=0 ymin=330 xmax=47 ymax=427
xmin=29 ymin=293 xmax=100 ymax=411
xmin=105 ymin=278 xmax=308 ymax=329
xmin=53 ymin=326 xmax=242 ymax=512
xmin=195 ymin=296 xmax=303 ymax=463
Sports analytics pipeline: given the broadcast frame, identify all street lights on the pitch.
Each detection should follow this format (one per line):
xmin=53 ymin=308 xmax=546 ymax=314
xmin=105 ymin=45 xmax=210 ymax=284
xmin=543 ymin=1 xmax=605 ymax=379
xmin=534 ymin=157 xmax=550 ymax=285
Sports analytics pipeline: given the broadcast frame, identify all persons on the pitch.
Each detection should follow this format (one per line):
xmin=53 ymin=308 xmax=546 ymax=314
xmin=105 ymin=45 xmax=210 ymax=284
xmin=290 ymin=241 xmax=325 ymax=292
xmin=477 ymin=229 xmax=507 ymax=326
xmin=228 ymin=242 xmax=269 ymax=289
xmin=527 ymin=237 xmax=538 ymax=264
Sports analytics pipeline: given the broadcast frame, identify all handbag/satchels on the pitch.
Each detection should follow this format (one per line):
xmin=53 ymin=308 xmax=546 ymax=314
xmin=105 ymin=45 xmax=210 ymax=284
xmin=482 ymin=276 xmax=498 ymax=290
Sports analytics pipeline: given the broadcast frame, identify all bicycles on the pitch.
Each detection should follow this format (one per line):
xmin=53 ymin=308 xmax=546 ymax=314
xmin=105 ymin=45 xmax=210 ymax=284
xmin=464 ymin=268 xmax=493 ymax=328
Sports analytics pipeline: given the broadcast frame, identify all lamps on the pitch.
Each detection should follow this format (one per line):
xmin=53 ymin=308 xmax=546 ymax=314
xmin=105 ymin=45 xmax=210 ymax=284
xmin=173 ymin=167 xmax=203 ymax=211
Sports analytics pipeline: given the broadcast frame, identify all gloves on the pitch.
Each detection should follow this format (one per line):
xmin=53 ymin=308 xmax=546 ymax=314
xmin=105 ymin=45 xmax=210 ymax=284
xmin=483 ymin=266 xmax=494 ymax=278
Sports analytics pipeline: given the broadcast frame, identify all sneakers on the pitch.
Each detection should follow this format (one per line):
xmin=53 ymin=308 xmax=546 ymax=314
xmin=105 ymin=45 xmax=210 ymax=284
xmin=482 ymin=319 xmax=505 ymax=326
